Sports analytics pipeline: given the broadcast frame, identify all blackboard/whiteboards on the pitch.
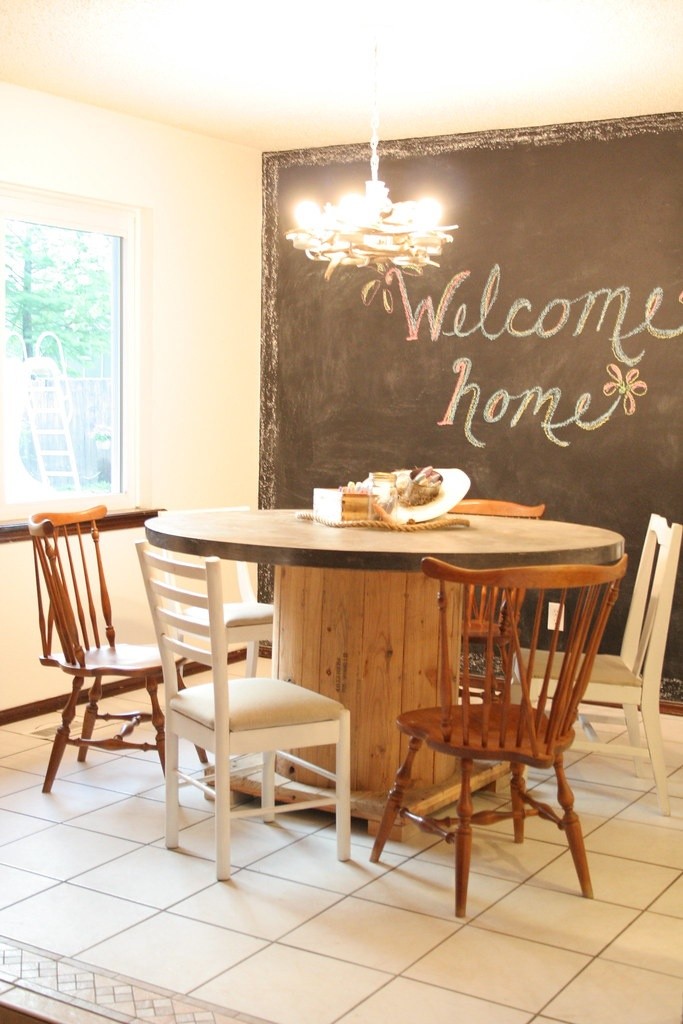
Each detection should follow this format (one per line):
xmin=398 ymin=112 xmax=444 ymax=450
xmin=256 ymin=112 xmax=682 ymax=717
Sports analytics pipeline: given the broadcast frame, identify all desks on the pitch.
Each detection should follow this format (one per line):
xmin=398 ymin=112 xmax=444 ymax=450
xmin=144 ymin=509 xmax=625 ymax=842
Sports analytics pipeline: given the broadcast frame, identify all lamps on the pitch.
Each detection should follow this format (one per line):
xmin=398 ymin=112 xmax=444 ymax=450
xmin=282 ymin=90 xmax=459 ymax=282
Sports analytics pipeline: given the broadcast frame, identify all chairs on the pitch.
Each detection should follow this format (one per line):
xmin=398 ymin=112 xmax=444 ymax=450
xmin=370 ymin=557 xmax=628 ymax=918
xmin=513 ymin=513 xmax=683 ymax=816
xmin=27 ymin=505 xmax=209 ymax=794
xmin=448 ymin=500 xmax=546 ymax=701
xmin=157 ymin=510 xmax=274 ymax=679
xmin=135 ymin=539 xmax=350 ymax=881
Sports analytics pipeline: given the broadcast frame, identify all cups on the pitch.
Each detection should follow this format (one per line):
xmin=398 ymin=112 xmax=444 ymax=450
xmin=404 ymin=468 xmax=443 ymax=505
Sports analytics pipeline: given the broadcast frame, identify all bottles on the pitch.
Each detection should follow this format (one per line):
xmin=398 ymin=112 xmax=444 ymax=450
xmin=367 ymin=472 xmax=398 ymax=524
